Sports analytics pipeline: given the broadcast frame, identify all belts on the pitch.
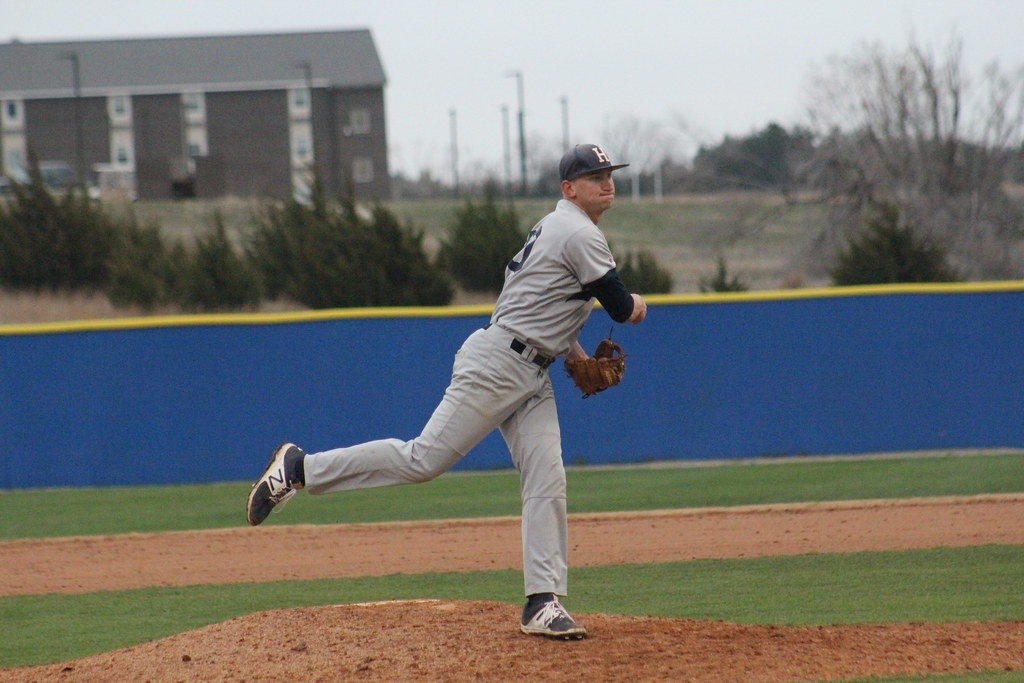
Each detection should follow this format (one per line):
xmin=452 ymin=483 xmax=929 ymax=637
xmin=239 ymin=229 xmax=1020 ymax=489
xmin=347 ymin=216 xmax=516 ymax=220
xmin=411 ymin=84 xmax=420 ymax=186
xmin=485 ymin=323 xmax=556 ymax=370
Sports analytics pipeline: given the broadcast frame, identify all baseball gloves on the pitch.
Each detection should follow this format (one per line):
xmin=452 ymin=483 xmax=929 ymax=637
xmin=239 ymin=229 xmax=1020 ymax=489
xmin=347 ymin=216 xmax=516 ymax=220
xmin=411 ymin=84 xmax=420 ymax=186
xmin=561 ymin=325 xmax=629 ymax=400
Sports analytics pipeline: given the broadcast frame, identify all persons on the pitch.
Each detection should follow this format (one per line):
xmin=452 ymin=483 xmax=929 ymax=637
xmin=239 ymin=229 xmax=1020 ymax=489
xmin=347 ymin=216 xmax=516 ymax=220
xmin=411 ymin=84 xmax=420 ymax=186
xmin=247 ymin=143 xmax=647 ymax=639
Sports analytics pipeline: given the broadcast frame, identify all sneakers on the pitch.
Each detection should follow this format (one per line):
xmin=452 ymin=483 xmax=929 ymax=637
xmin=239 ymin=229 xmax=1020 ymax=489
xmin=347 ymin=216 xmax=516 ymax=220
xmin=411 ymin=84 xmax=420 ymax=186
xmin=245 ymin=443 xmax=305 ymax=526
xmin=520 ymin=592 xmax=587 ymax=639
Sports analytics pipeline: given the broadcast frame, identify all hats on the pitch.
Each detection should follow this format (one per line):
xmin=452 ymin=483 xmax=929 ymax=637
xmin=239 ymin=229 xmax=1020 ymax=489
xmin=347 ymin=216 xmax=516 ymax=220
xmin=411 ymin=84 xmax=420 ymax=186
xmin=559 ymin=143 xmax=630 ymax=183
